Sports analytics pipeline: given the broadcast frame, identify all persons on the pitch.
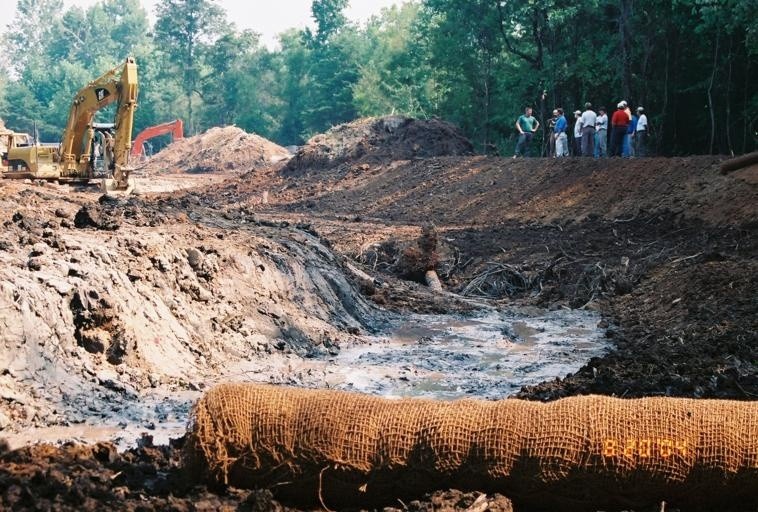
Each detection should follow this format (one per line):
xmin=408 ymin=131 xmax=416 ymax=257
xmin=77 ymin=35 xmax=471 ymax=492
xmin=609 ymin=102 xmax=630 ymax=159
xmin=628 ymin=115 xmax=638 ymax=157
xmin=620 ymin=100 xmax=633 ymax=158
xmin=545 ymin=108 xmax=560 ymax=158
xmin=554 ymin=108 xmax=570 ymax=159
xmin=511 ymin=107 xmax=540 ymax=159
xmin=573 ymin=110 xmax=584 ymax=156
xmin=635 ymin=107 xmax=649 ymax=157
xmin=578 ymin=101 xmax=597 ymax=157
xmin=594 ymin=106 xmax=608 ymax=158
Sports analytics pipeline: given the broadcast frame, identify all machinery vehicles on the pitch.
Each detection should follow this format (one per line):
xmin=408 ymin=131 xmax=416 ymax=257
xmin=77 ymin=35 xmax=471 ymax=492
xmin=0 ymin=57 xmax=183 ymax=196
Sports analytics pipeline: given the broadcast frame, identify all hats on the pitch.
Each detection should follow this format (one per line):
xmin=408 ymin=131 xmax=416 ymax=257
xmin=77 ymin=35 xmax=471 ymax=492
xmin=552 ymin=108 xmax=563 ymax=116
xmin=635 ymin=106 xmax=645 ymax=112
xmin=597 ymin=105 xmax=607 ymax=112
xmin=617 ymin=100 xmax=627 ymax=108
xmin=573 ymin=110 xmax=582 ymax=115
